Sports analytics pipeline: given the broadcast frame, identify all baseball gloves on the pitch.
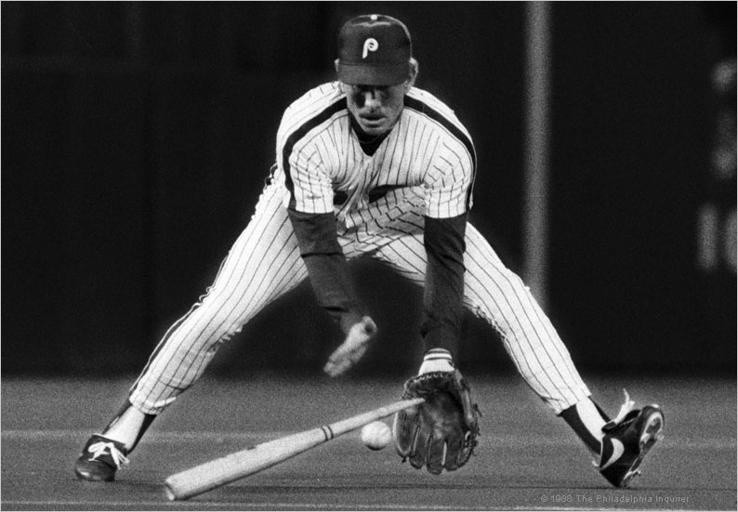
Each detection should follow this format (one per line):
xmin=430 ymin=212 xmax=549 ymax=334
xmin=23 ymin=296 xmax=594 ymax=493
xmin=393 ymin=349 xmax=482 ymax=473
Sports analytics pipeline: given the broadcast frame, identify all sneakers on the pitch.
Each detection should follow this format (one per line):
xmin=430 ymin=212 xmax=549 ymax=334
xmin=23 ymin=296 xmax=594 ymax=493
xmin=593 ymin=404 xmax=663 ymax=486
xmin=76 ymin=435 xmax=130 ymax=480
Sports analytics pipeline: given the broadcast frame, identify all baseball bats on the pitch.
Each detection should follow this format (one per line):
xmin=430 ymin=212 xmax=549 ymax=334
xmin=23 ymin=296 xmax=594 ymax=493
xmin=165 ymin=399 xmax=423 ymax=500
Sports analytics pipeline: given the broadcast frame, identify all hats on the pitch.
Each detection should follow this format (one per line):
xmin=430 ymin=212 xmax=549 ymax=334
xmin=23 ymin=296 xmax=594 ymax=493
xmin=338 ymin=15 xmax=411 ymax=86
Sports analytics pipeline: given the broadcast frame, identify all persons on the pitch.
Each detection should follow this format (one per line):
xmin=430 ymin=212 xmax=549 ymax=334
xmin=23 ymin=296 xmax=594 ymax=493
xmin=75 ymin=15 xmax=665 ymax=490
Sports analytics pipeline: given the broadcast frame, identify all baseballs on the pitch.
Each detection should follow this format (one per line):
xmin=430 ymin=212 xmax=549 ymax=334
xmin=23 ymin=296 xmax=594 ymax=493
xmin=361 ymin=420 xmax=390 ymax=450
